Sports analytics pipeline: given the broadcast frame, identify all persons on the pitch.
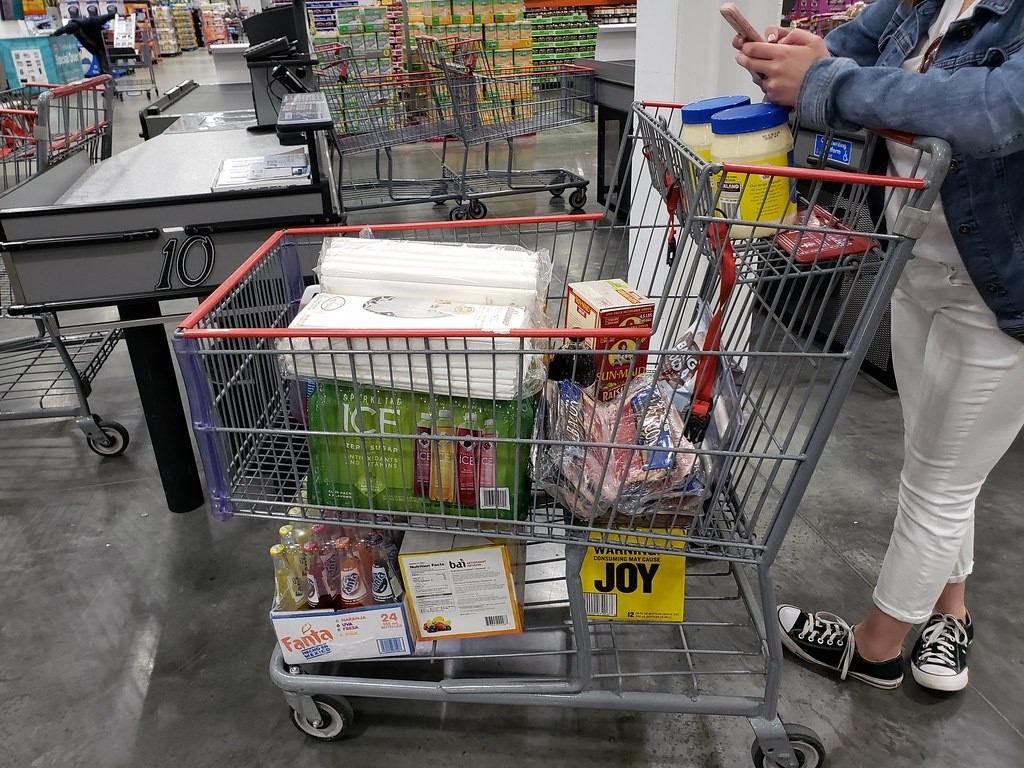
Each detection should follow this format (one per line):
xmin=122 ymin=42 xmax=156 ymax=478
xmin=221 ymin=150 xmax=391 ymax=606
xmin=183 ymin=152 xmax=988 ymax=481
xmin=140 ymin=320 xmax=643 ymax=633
xmin=732 ymin=0 xmax=1024 ymax=693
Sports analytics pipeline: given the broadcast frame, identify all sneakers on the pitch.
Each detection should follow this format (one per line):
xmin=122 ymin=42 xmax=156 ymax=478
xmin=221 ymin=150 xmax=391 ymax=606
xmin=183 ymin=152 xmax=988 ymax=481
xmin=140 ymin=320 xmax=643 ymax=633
xmin=777 ymin=604 xmax=903 ymax=689
xmin=911 ymin=606 xmax=974 ymax=691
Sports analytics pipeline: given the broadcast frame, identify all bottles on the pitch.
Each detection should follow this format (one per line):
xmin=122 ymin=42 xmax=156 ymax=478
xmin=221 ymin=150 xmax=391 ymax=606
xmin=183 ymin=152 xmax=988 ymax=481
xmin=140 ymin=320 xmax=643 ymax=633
xmin=550 ymin=328 xmax=595 ymax=407
xmin=457 ymin=414 xmax=479 ymax=507
xmin=270 ymin=475 xmax=404 ymax=612
xmin=429 ymin=410 xmax=455 ymax=503
xmin=413 ymin=413 xmax=431 ymax=497
xmin=479 ymin=419 xmax=496 ymax=503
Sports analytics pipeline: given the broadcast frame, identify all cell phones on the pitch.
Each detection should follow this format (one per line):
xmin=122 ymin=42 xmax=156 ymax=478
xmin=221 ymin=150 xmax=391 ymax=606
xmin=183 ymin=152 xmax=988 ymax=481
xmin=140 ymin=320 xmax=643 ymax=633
xmin=720 ymin=3 xmax=765 ymax=43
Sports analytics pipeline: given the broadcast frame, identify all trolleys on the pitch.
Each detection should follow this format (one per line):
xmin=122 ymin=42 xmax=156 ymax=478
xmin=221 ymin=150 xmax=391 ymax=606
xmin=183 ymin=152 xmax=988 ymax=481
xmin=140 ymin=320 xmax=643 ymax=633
xmin=162 ymin=95 xmax=947 ymax=768
xmin=312 ymin=30 xmax=596 ymax=221
xmin=0 ymin=75 xmax=132 ymax=458
xmin=100 ymin=28 xmax=160 ymax=99
xmin=226 ymin=19 xmax=245 ymax=45
